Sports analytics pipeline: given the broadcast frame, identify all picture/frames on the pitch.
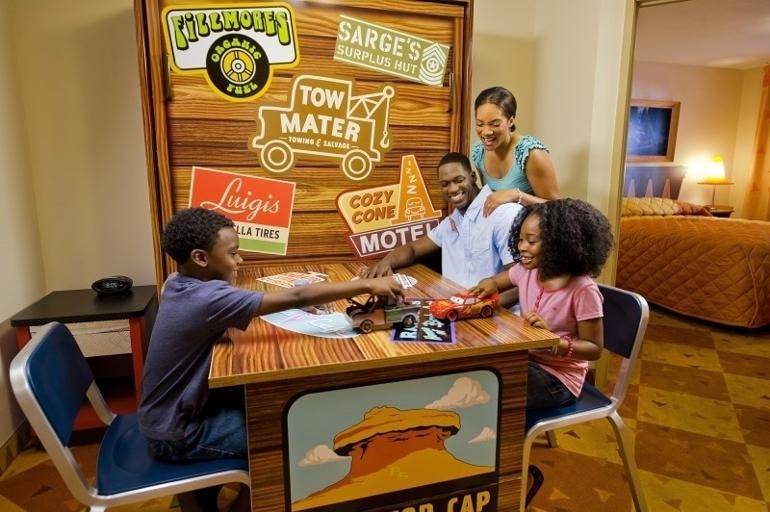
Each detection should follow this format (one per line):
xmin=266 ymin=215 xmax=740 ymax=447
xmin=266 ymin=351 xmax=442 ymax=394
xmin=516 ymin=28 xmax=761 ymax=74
xmin=625 ymin=97 xmax=682 ymax=163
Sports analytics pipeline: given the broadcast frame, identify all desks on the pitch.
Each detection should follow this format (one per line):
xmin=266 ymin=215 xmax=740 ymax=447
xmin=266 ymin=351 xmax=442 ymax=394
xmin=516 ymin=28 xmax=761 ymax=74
xmin=201 ymin=257 xmax=563 ymax=510
xmin=10 ymin=284 xmax=160 ymax=446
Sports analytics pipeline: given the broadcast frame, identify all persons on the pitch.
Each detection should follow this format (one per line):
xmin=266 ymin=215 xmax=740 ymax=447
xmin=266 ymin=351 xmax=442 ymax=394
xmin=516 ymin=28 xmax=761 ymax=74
xmin=137 ymin=207 xmax=401 ymax=512
xmin=452 ymin=86 xmax=560 ymax=212
xmin=481 ymin=197 xmax=612 ymax=509
xmin=364 ymin=151 xmax=530 ymax=320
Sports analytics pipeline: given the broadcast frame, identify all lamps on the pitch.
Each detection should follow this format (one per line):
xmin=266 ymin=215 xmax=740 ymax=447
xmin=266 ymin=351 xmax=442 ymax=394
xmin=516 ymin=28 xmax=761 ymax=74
xmin=696 ymin=156 xmax=736 ymax=207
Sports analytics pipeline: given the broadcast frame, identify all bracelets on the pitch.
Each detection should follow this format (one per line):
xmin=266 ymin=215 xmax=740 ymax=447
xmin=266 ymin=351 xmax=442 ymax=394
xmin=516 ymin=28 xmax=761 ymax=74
xmin=562 ymin=336 xmax=573 ymax=361
xmin=550 ymin=341 xmax=559 ymax=354
xmin=516 ymin=187 xmax=523 ymax=204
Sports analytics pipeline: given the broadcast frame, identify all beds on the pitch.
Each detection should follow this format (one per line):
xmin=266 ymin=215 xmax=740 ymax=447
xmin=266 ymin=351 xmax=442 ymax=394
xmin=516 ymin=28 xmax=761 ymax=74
xmin=616 ymin=167 xmax=769 ymax=331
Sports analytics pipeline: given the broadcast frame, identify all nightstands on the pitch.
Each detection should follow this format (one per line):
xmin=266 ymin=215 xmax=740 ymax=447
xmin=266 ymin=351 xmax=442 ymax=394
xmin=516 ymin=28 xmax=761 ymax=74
xmin=704 ymin=204 xmax=734 ymax=217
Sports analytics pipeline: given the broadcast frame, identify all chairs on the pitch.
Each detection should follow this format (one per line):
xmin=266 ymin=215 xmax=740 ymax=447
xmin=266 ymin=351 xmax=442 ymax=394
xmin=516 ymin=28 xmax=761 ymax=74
xmin=8 ymin=320 xmax=251 ymax=512
xmin=523 ymin=283 xmax=652 ymax=512
